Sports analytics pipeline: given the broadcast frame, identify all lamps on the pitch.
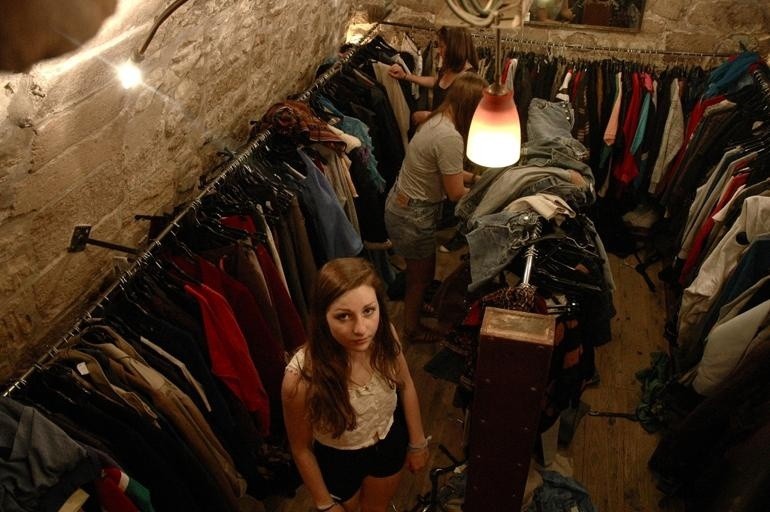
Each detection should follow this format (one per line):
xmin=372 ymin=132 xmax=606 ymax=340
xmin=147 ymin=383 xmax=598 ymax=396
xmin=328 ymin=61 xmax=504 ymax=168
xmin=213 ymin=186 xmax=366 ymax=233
xmin=466 ymin=14 xmax=524 ymax=170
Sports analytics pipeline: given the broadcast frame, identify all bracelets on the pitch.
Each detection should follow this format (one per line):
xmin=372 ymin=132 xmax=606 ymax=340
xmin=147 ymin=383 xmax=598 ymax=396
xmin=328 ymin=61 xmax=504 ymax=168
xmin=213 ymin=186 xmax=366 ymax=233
xmin=403 ymin=72 xmax=409 ymax=81
xmin=316 ymin=500 xmax=341 ymax=512
xmin=471 ymin=173 xmax=477 ymax=185
xmin=408 ymin=433 xmax=431 ymax=451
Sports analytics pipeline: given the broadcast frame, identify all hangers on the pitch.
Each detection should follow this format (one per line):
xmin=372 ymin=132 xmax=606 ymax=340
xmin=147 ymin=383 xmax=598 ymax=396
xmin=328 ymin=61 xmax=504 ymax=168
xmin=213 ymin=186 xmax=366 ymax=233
xmin=753 ymin=68 xmax=769 ymax=97
xmin=477 ymin=25 xmax=734 ymax=74
xmin=517 ymin=209 xmax=610 ymax=319
xmin=0 ymin=20 xmax=439 ymax=463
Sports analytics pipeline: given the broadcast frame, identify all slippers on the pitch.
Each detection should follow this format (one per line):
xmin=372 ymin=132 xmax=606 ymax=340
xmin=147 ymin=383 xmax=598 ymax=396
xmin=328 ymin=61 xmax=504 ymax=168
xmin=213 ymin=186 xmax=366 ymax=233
xmin=420 ymin=298 xmax=440 ymax=319
xmin=401 ymin=325 xmax=440 ymax=342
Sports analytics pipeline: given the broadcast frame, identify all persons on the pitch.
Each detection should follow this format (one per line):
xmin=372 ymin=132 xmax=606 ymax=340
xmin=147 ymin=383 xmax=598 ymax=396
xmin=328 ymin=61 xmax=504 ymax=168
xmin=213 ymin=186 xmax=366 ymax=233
xmin=384 ymin=73 xmax=495 ymax=344
xmin=389 ymin=26 xmax=481 ymax=127
xmin=281 ymin=257 xmax=432 ymax=512
